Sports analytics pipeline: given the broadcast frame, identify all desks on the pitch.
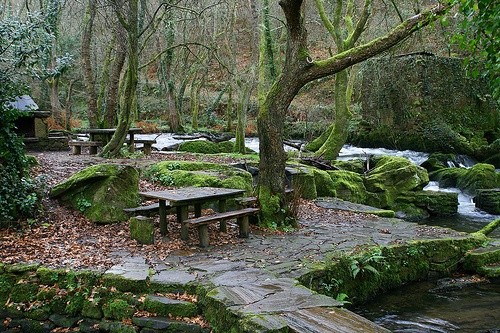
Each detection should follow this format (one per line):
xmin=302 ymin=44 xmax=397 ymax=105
xmin=81 ymin=129 xmax=143 ymax=153
xmin=138 ymin=187 xmax=247 ymax=241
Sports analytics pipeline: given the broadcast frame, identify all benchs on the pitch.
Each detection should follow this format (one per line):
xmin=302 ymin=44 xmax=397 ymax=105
xmin=123 ymin=201 xmax=172 ymax=228
xmin=68 ymin=141 xmax=102 ymax=155
xmin=183 ymin=207 xmax=261 ymax=249
xmin=125 ymin=140 xmax=157 ymax=155
